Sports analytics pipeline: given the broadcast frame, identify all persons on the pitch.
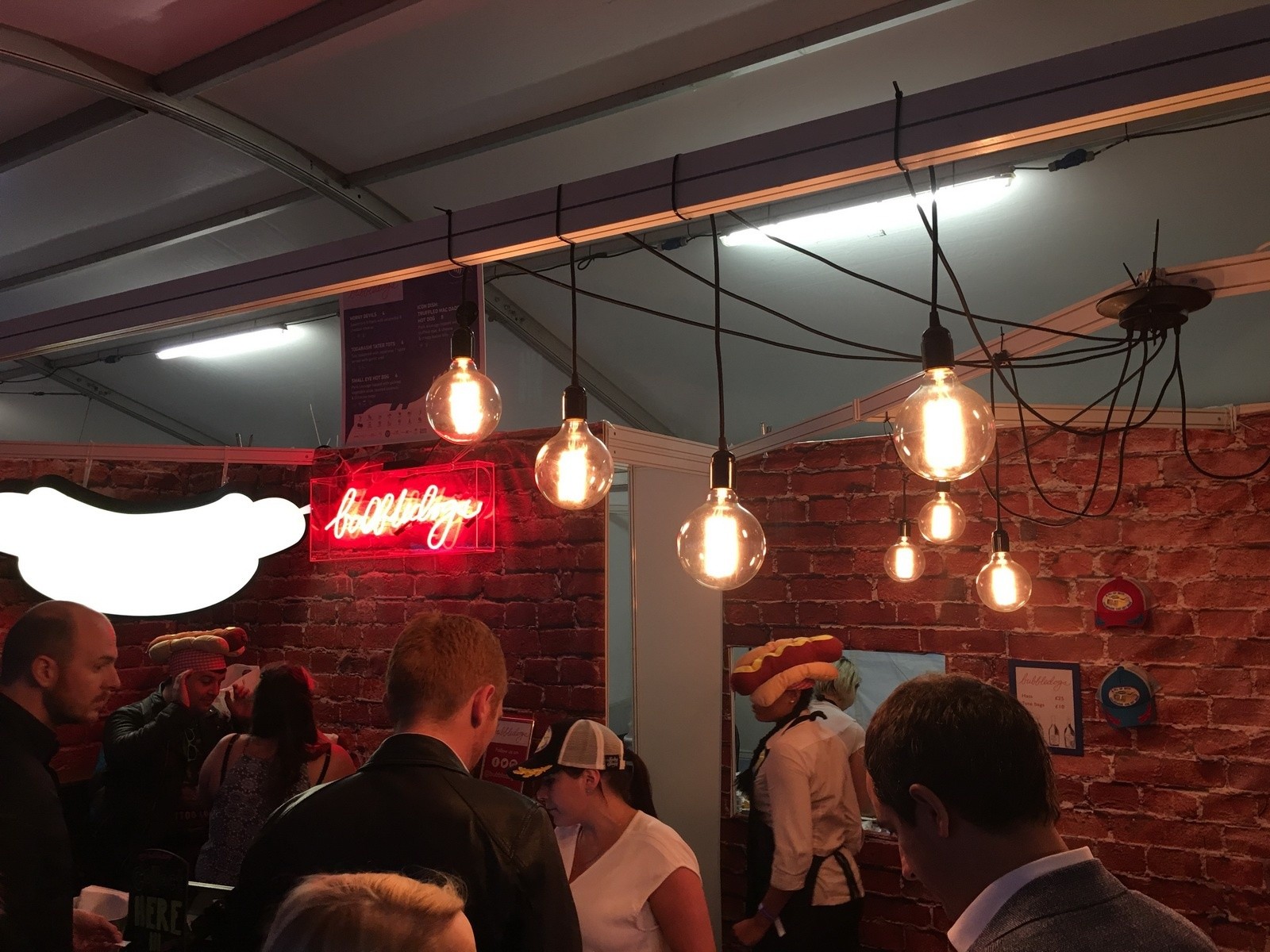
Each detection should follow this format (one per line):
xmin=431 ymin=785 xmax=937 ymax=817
xmin=261 ymin=872 xmax=477 ymax=952
xmin=866 ymin=673 xmax=1218 ymax=952
xmin=730 ymin=633 xmax=868 ymax=952
xmin=808 ymin=656 xmax=871 ymax=814
xmin=92 ymin=627 xmax=254 ymax=952
xmin=507 ymin=720 xmax=716 ymax=952
xmin=237 ymin=612 xmax=584 ymax=952
xmin=196 ymin=658 xmax=356 ymax=885
xmin=0 ymin=600 xmax=123 ymax=952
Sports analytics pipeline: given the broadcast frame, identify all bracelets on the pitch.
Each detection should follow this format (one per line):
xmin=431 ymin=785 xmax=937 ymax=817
xmin=757 ymin=902 xmax=776 ymax=922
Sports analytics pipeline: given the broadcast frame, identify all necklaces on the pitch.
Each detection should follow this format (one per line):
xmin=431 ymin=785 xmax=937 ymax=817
xmin=574 ymin=828 xmax=607 ymax=871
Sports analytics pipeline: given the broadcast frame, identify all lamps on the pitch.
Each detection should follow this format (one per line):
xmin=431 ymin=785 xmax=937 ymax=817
xmin=719 ymin=163 xmax=1018 ymax=248
xmin=153 ymin=323 xmax=288 ymax=361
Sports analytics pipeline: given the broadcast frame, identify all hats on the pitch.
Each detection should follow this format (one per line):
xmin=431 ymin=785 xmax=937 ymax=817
xmin=730 ymin=636 xmax=843 ymax=706
xmin=147 ymin=625 xmax=249 ymax=678
xmin=507 ymin=717 xmax=627 ymax=780
